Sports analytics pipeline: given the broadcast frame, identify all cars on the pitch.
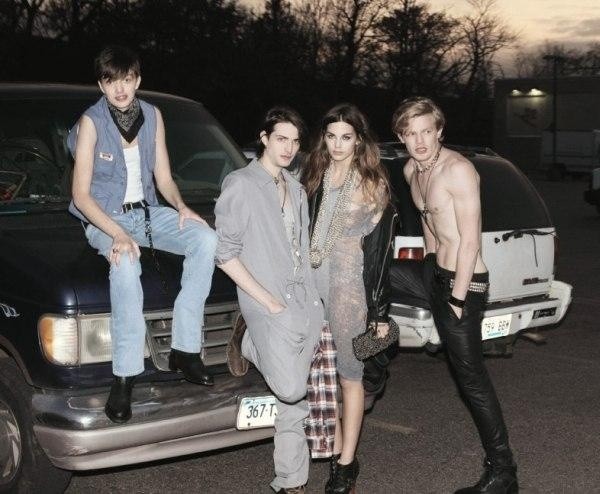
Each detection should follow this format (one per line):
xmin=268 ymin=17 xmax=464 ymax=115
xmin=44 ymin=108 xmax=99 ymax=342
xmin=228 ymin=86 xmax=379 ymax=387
xmin=164 ymin=143 xmax=581 ymax=356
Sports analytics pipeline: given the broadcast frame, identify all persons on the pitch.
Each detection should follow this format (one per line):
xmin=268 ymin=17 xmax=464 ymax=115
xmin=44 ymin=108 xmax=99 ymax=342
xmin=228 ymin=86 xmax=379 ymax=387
xmin=63 ymin=41 xmax=220 ymax=426
xmin=215 ymin=106 xmax=328 ymax=493
xmin=302 ymin=102 xmax=394 ymax=492
xmin=381 ymin=92 xmax=525 ymax=494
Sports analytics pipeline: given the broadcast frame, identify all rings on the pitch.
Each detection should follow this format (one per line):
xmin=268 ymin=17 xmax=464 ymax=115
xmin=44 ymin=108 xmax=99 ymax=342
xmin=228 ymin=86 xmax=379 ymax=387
xmin=112 ymin=247 xmax=119 ymax=253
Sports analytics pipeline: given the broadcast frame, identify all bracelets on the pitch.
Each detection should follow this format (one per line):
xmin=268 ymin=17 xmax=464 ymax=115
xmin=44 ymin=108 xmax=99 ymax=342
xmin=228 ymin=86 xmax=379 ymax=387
xmin=446 ymin=295 xmax=466 ymax=309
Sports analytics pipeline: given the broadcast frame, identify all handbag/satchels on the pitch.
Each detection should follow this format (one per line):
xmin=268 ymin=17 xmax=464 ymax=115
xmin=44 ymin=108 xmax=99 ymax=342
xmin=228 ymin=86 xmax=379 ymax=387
xmin=351 ymin=317 xmax=400 ymax=361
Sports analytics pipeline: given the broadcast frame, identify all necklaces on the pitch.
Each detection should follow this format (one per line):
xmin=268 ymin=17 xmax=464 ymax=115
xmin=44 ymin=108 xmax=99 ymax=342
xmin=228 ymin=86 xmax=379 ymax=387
xmin=412 ymin=143 xmax=444 ymax=220
xmin=307 ymin=160 xmax=358 ymax=269
xmin=271 ymin=172 xmax=290 ymax=220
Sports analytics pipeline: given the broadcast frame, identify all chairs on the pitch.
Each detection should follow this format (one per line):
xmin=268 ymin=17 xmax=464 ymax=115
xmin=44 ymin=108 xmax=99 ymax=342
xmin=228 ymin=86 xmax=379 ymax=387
xmin=4 ymin=137 xmax=55 ymax=187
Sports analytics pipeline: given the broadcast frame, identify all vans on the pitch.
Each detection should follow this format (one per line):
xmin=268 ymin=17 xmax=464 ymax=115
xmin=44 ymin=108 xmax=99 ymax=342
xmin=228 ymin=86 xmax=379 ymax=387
xmin=2 ymin=70 xmax=384 ymax=480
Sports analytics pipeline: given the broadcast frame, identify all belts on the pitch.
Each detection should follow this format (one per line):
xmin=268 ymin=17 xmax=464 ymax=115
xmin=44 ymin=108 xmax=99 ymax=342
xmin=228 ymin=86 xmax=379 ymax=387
xmin=122 ymin=199 xmax=149 ymax=213
xmin=433 ymin=268 xmax=491 ymax=294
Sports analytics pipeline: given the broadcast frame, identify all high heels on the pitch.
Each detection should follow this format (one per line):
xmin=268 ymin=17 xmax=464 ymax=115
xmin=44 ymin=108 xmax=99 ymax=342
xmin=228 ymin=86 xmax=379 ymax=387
xmin=326 ymin=454 xmax=358 ymax=494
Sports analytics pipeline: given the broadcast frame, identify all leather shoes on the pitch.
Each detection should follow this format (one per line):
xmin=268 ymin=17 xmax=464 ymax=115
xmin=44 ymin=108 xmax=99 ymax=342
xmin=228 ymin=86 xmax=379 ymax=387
xmin=454 ymin=457 xmax=519 ymax=494
xmin=169 ymin=350 xmax=214 ymax=385
xmin=226 ymin=311 xmax=250 ymax=377
xmin=105 ymin=374 xmax=132 ymax=423
xmin=364 ymin=352 xmax=390 ymax=392
